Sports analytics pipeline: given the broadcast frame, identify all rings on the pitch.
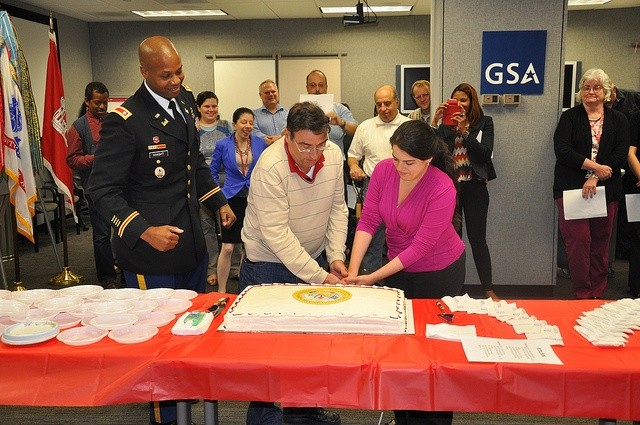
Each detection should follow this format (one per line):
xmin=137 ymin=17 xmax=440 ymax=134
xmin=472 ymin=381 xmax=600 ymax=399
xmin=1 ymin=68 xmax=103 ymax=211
xmin=460 ymin=114 xmax=465 ymax=118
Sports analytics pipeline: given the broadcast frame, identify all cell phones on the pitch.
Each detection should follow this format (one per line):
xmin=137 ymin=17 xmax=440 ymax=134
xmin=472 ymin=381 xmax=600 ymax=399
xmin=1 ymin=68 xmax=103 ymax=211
xmin=442 ymin=98 xmax=460 ymax=126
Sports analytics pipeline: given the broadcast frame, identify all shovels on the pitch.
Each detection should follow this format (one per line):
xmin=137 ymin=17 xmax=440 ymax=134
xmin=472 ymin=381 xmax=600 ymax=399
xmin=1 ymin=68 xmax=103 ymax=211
xmin=437 ymin=301 xmax=453 ymax=323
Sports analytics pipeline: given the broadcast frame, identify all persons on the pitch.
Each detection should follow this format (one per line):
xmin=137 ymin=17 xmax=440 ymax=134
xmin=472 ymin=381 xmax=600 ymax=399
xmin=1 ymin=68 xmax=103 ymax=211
xmin=628 ymin=93 xmax=640 ymax=297
xmin=195 ymin=91 xmax=235 ymax=285
xmin=238 ymin=101 xmax=348 ymax=425
xmin=430 ymin=82 xmax=497 ymax=299
xmin=293 ymin=70 xmax=360 ymax=209
xmin=210 ymin=108 xmax=267 ymax=293
xmin=65 ymin=81 xmax=127 ymax=289
xmin=347 ymin=85 xmax=415 ymax=288
xmin=552 ymin=68 xmax=626 ymax=299
xmin=250 ymin=79 xmax=290 ymax=146
xmin=85 ymin=34 xmax=235 ymax=425
xmin=343 ymin=119 xmax=465 ymax=425
xmin=407 ymin=79 xmax=431 ymax=127
xmin=78 ymin=102 xmax=89 ymax=118
xmin=605 ymin=77 xmax=637 ymax=260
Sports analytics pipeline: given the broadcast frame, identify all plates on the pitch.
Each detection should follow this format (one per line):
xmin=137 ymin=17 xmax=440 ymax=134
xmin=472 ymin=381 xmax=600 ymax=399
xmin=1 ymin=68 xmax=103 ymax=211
xmin=86 ymin=300 xmax=135 ymax=315
xmin=6 ymin=307 xmax=61 ymax=323
xmin=105 ymin=286 xmax=145 ymax=303
xmin=107 ymin=323 xmax=160 ymax=345
xmin=174 ymin=288 xmax=199 ymax=301
xmin=77 ymin=315 xmax=99 ymax=330
xmin=58 ymin=284 xmax=105 ymax=297
xmin=0 ymin=322 xmax=8 ymax=341
xmin=0 ymin=289 xmax=11 ymax=300
xmin=12 ymin=289 xmax=25 ymax=302
xmin=153 ymin=297 xmax=193 ymax=316
xmin=33 ymin=294 xmax=86 ymax=313
xmin=65 ymin=301 xmax=88 ymax=319
xmin=47 ymin=311 xmax=82 ymax=330
xmin=0 ymin=300 xmax=31 ymax=319
xmin=145 ymin=286 xmax=177 ymax=300
xmin=133 ymin=308 xmax=177 ymax=327
xmin=1 ymin=320 xmax=61 ymax=346
xmin=131 ymin=298 xmax=159 ymax=314
xmin=87 ymin=297 xmax=105 ymax=303
xmin=89 ymin=313 xmax=139 ymax=330
xmin=25 ymin=288 xmax=57 ymax=301
xmin=54 ymin=325 xmax=108 ymax=347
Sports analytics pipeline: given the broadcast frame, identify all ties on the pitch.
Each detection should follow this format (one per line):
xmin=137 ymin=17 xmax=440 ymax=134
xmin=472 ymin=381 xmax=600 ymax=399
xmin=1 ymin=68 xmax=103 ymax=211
xmin=166 ymin=100 xmax=186 ymax=124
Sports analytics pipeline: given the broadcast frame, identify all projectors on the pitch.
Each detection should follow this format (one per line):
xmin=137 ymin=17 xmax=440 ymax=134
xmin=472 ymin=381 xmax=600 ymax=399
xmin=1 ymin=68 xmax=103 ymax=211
xmin=342 ymin=0 xmax=377 ymax=27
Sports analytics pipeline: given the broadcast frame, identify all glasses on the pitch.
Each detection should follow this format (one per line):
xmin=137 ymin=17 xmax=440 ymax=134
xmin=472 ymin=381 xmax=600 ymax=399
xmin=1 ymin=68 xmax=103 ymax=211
xmin=306 ymin=81 xmax=326 ymax=89
xmin=582 ymin=85 xmax=603 ymax=92
xmin=292 ymin=131 xmax=333 ymax=153
xmin=413 ymin=92 xmax=430 ymax=99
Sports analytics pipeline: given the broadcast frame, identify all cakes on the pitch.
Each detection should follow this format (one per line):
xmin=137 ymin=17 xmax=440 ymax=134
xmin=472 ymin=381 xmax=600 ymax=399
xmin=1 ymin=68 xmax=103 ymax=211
xmin=223 ymin=283 xmax=407 ymax=332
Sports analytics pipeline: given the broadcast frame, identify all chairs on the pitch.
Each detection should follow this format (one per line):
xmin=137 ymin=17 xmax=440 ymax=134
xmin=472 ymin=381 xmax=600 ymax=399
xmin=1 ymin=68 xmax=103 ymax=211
xmin=42 ymin=182 xmax=82 ymax=240
xmin=31 ymin=186 xmax=59 ymax=253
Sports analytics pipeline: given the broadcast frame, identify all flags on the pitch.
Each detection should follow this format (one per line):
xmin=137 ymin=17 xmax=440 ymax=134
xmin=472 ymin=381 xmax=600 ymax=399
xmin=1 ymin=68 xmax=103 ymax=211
xmin=6 ymin=14 xmax=43 ymax=176
xmin=0 ymin=32 xmax=38 ymax=245
xmin=41 ymin=31 xmax=81 ymax=225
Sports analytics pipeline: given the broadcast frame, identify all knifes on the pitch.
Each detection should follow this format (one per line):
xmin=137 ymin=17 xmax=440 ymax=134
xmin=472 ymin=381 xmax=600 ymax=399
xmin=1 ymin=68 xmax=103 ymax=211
xmin=208 ymin=297 xmax=229 ymax=318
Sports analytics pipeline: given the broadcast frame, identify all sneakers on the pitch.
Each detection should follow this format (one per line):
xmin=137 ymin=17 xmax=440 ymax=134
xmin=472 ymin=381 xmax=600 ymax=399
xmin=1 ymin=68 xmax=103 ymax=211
xmin=284 ymin=409 xmax=340 ymax=424
